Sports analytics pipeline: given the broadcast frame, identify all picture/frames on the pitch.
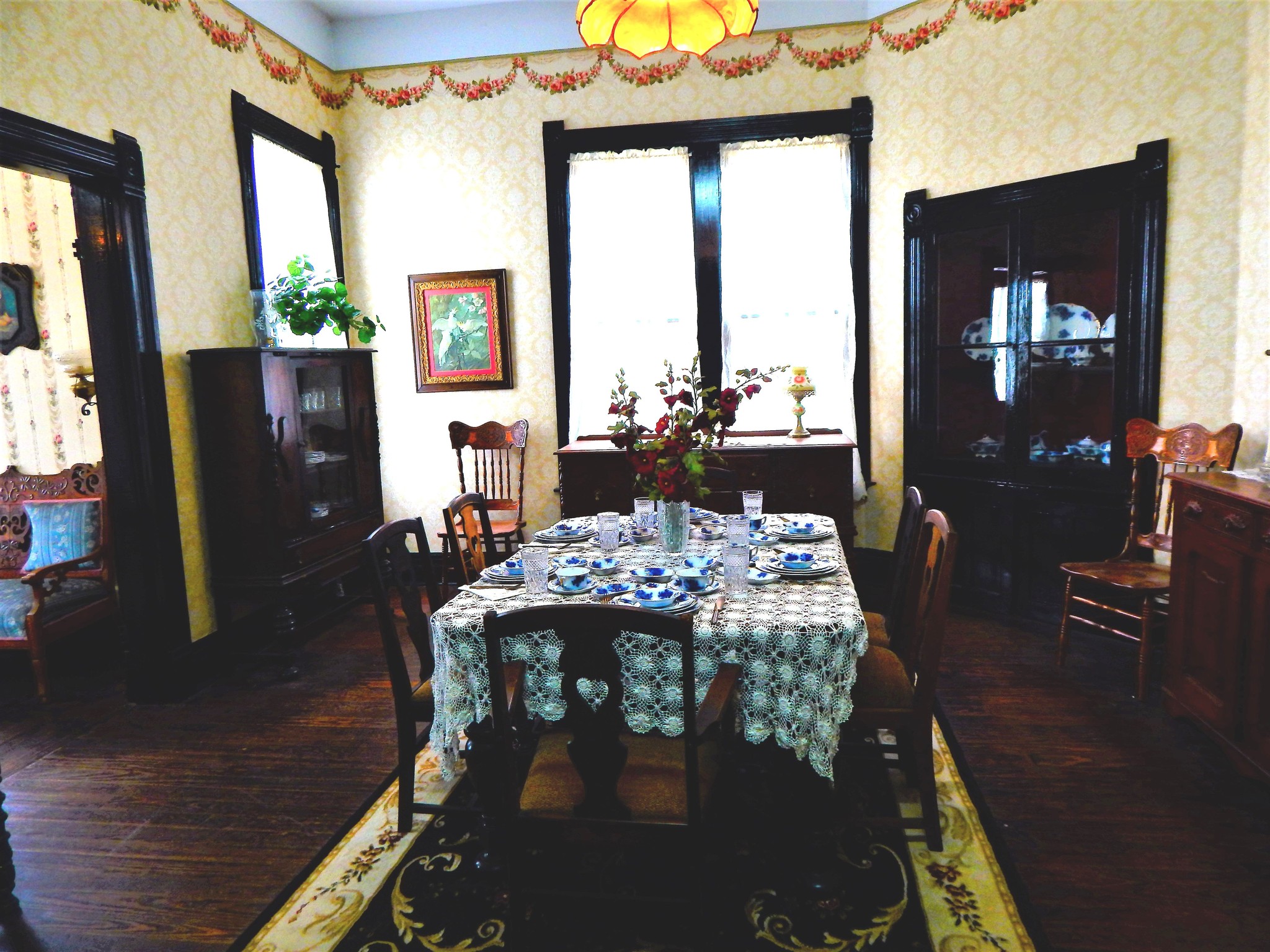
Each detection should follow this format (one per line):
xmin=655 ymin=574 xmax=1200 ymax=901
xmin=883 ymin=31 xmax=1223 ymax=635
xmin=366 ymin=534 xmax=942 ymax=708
xmin=408 ymin=269 xmax=515 ymax=393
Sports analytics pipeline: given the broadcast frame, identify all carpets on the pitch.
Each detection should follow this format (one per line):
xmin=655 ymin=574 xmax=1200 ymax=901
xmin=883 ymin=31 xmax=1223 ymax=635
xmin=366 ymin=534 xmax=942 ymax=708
xmin=229 ymin=710 xmax=1056 ymax=952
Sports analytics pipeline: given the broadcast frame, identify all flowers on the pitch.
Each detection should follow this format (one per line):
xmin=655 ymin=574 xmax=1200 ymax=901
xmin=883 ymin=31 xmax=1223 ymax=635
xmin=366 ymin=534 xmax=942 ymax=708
xmin=607 ymin=351 xmax=791 ymax=502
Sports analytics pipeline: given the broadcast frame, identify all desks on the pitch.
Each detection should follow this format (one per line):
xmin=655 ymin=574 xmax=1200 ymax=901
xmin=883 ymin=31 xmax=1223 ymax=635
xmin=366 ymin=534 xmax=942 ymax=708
xmin=427 ymin=513 xmax=868 ymax=788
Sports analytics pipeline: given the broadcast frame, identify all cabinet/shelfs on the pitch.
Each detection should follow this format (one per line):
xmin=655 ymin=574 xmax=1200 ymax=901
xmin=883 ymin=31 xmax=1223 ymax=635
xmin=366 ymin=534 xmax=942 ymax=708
xmin=901 ymin=137 xmax=1169 ymax=632
xmin=553 ymin=428 xmax=857 ymax=574
xmin=185 ymin=348 xmax=385 ymax=678
xmin=1158 ymin=471 xmax=1269 ymax=785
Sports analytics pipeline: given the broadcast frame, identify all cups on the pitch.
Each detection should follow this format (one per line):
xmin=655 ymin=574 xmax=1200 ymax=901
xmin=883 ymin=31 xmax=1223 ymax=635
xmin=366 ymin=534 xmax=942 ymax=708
xmin=722 ymin=543 xmax=748 ymax=593
xmin=597 ymin=512 xmax=620 ymax=551
xmin=748 ymin=515 xmax=767 ymax=531
xmin=726 ymin=518 xmax=749 ymax=545
xmin=966 ymin=432 xmax=1112 ymax=466
xmin=300 ymin=387 xmax=341 ymax=410
xmin=742 ymin=490 xmax=763 ymax=519
xmin=675 ymin=568 xmax=714 ymax=592
xmin=634 ymin=497 xmax=654 ymax=528
xmin=521 ymin=547 xmax=548 ymax=594
xmin=749 ymin=543 xmax=758 ymax=561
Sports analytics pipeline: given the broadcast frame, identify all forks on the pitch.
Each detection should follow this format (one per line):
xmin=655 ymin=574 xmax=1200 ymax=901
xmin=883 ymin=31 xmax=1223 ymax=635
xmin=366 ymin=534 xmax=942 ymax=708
xmin=601 ymin=593 xmax=612 ymax=605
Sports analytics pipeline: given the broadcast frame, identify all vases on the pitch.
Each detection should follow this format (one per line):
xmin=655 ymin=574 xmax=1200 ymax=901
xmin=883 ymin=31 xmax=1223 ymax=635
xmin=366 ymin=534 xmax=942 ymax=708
xmin=249 ymin=289 xmax=285 ymax=348
xmin=656 ymin=499 xmax=691 ymax=555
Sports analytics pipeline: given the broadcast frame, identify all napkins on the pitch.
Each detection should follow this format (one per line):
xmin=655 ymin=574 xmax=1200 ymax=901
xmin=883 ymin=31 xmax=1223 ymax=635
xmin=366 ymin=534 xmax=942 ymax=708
xmin=457 ymin=584 xmax=526 ymax=601
xmin=518 ymin=541 xmax=592 ymax=554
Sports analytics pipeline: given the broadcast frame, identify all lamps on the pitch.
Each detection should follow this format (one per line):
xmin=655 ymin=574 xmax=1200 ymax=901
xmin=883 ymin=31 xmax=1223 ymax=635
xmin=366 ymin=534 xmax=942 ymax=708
xmin=576 ymin=0 xmax=757 ymax=59
xmin=57 ymin=347 xmax=98 ymax=416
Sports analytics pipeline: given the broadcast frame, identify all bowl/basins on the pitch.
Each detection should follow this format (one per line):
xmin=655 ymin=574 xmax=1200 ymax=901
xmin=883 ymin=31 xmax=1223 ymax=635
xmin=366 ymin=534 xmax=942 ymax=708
xmin=551 ymin=524 xmax=583 ymax=536
xmin=499 ymin=560 xmax=525 ymax=575
xmin=783 ymin=520 xmax=816 ymax=535
xmin=627 ymin=528 xmax=658 ymax=541
xmin=587 ymin=557 xmax=622 ymax=576
xmin=555 ymin=566 xmax=591 ymax=591
xmin=689 ymin=507 xmax=702 ymax=518
xmin=777 ymin=550 xmax=819 ymax=569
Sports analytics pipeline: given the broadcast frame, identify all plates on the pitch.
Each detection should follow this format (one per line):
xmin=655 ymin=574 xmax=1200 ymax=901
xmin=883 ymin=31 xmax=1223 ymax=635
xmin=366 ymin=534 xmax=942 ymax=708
xmin=308 ymin=500 xmax=333 ymax=519
xmin=698 ymin=524 xmax=726 ymax=540
xmin=481 ymin=560 xmax=562 ymax=582
xmin=552 ymin=556 xmax=587 ymax=569
xmin=482 ymin=504 xmax=837 ymax=615
xmin=304 ymin=448 xmax=349 ymax=467
xmin=960 ymin=300 xmax=1118 ymax=366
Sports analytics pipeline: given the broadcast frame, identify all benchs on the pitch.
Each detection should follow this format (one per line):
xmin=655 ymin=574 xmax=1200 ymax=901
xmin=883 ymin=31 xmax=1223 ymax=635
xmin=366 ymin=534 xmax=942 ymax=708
xmin=0 ymin=456 xmax=116 ymax=702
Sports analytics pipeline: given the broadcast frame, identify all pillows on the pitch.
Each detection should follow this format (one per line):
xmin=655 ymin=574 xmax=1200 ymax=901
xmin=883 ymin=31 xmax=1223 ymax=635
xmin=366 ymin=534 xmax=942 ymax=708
xmin=22 ymin=499 xmax=103 ymax=572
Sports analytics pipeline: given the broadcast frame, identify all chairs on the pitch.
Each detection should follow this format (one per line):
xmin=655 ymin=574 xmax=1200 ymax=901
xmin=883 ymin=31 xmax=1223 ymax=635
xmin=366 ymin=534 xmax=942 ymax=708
xmin=859 ymin=483 xmax=927 ymax=770
xmin=1059 ymin=419 xmax=1242 ymax=701
xmin=474 ymin=603 xmax=743 ymax=950
xmin=839 ymin=509 xmax=954 ymax=852
xmin=438 ymin=418 xmax=528 ymax=599
xmin=359 ymin=516 xmax=488 ymax=833
xmin=442 ymin=493 xmax=498 ymax=591
